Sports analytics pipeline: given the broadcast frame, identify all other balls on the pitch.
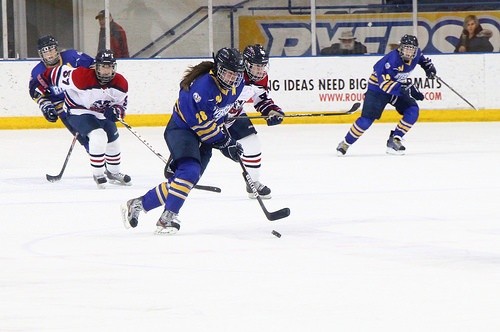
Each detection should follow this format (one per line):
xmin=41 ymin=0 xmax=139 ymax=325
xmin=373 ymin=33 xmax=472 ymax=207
xmin=271 ymin=230 xmax=282 ymax=238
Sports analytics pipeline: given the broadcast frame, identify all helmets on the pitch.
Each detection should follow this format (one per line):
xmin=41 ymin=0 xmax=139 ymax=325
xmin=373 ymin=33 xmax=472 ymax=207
xmin=243 ymin=44 xmax=269 ymax=82
xmin=399 ymin=34 xmax=418 ymax=62
xmin=96 ymin=51 xmax=117 ymax=85
xmin=214 ymin=47 xmax=245 ymax=91
xmin=37 ymin=36 xmax=61 ymax=67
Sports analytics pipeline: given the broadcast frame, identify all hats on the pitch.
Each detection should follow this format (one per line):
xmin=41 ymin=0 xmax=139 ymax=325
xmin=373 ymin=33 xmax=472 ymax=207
xmin=338 ymin=31 xmax=356 ymax=41
xmin=96 ymin=10 xmax=111 ymax=19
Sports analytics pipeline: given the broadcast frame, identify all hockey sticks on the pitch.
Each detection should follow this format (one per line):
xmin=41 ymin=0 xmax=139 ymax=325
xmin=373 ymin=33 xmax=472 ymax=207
xmin=429 ymin=71 xmax=488 ymax=110
xmin=222 ymin=118 xmax=291 ymax=221
xmin=229 ymin=103 xmax=363 ymax=118
xmin=108 ymin=105 xmax=221 ymax=193
xmin=45 ymin=131 xmax=78 ymax=181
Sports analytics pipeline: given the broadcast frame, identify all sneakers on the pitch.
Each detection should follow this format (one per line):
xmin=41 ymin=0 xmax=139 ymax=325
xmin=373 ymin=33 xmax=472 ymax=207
xmin=106 ymin=171 xmax=131 ymax=185
xmin=92 ymin=174 xmax=107 ymax=188
xmin=337 ymin=140 xmax=348 ymax=157
xmin=121 ymin=196 xmax=144 ymax=228
xmin=246 ymin=181 xmax=272 ymax=199
xmin=155 ymin=211 xmax=180 ymax=235
xmin=386 ymin=130 xmax=406 ymax=155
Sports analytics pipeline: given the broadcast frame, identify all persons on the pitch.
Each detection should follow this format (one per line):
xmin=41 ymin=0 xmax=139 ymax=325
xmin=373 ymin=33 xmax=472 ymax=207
xmin=124 ymin=45 xmax=249 ymax=236
xmin=28 ymin=34 xmax=99 ymax=155
xmin=335 ymin=33 xmax=436 ymax=156
xmin=28 ymin=48 xmax=132 ymax=188
xmin=94 ymin=9 xmax=130 ymax=58
xmin=319 ymin=28 xmax=368 ymax=56
xmin=454 ymin=14 xmax=496 ymax=53
xmin=162 ymin=44 xmax=286 ymax=200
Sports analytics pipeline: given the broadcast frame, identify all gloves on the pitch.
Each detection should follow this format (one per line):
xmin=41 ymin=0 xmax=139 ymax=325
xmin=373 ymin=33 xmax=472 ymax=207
xmin=422 ymin=58 xmax=436 ymax=79
xmin=30 ymin=84 xmax=49 ymax=98
xmin=219 ymin=137 xmax=243 ymax=162
xmin=104 ymin=107 xmax=120 ymax=122
xmin=259 ymin=100 xmax=283 ymax=126
xmin=43 ymin=106 xmax=58 ymax=123
xmin=408 ymin=84 xmax=423 ymax=101
